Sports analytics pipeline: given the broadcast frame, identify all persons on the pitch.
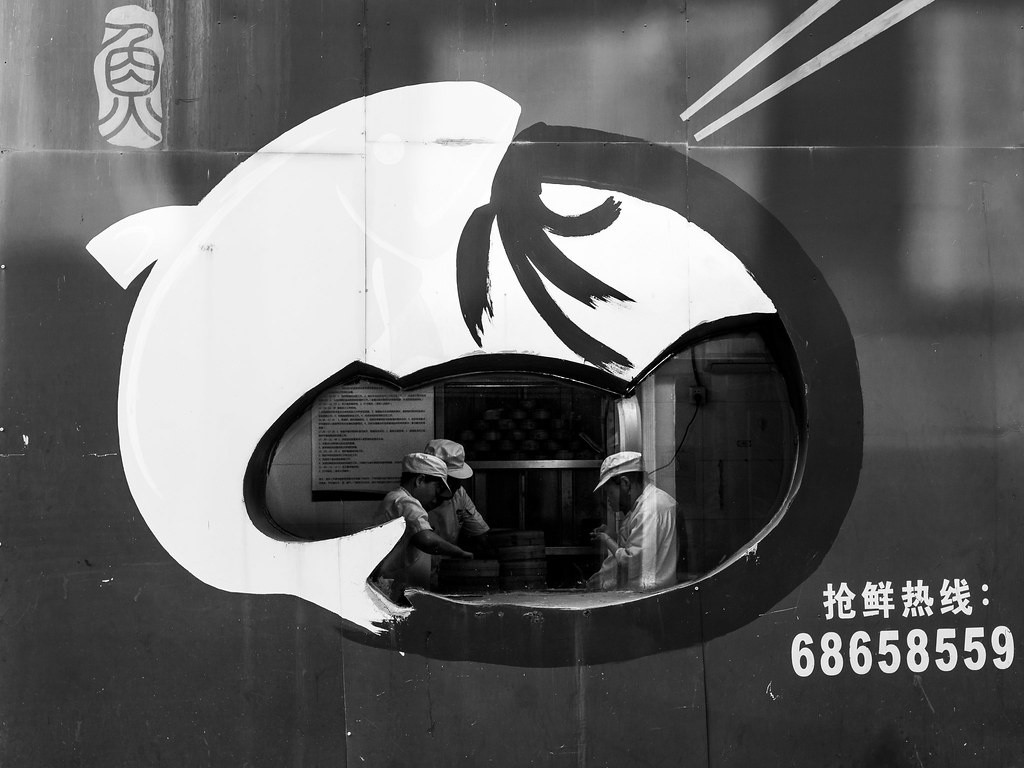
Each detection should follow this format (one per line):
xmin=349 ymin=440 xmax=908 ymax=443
xmin=377 ymin=438 xmax=489 ymax=607
xmin=588 ymin=450 xmax=679 ymax=595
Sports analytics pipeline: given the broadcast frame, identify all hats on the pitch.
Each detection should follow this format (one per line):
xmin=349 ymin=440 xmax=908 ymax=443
xmin=424 ymin=439 xmax=473 ymax=479
xmin=402 ymin=453 xmax=452 ymax=498
xmin=593 ymin=451 xmax=642 ymax=493
